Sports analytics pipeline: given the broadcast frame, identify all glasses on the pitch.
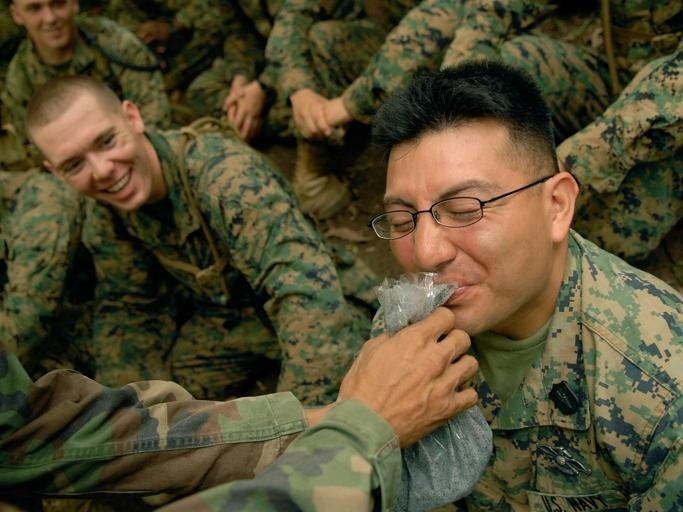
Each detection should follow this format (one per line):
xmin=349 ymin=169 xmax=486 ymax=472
xmin=368 ymin=175 xmax=554 ymax=240
